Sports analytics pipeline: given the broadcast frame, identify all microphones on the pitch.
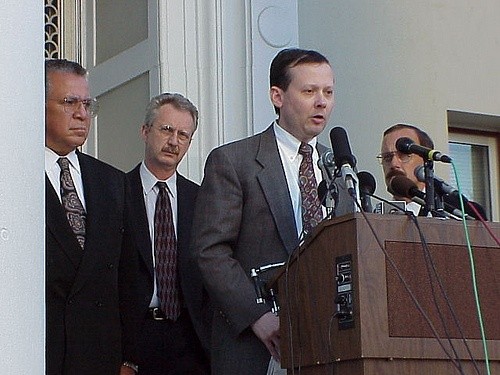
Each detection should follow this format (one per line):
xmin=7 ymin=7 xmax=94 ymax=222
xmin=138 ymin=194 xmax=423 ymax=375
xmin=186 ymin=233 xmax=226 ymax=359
xmin=390 ymin=137 xmax=477 ymax=221
xmin=318 ymin=127 xmax=376 ymax=214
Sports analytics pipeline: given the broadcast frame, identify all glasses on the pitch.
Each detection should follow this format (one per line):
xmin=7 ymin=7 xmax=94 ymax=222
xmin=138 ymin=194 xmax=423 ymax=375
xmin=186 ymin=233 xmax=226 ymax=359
xmin=376 ymin=150 xmax=415 ymax=165
xmin=147 ymin=124 xmax=194 ymax=145
xmin=48 ymin=95 xmax=102 ymax=119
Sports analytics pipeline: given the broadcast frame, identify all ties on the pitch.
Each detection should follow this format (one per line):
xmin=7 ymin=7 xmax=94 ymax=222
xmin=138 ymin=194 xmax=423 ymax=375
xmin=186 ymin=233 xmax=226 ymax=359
xmin=56 ymin=157 xmax=87 ymax=252
xmin=297 ymin=142 xmax=324 ymax=242
xmin=153 ymin=181 xmax=183 ymax=324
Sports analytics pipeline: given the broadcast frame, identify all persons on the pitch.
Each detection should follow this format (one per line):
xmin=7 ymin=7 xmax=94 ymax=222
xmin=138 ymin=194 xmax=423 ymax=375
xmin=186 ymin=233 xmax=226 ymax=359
xmin=377 ymin=124 xmax=488 ymax=221
xmin=118 ymin=93 xmax=212 ymax=375
xmin=46 ymin=59 xmax=148 ymax=375
xmin=193 ymin=49 xmax=356 ymax=375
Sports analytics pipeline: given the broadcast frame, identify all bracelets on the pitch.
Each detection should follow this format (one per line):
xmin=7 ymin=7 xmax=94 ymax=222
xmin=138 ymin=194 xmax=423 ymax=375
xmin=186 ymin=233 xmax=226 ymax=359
xmin=122 ymin=362 xmax=138 ymax=372
